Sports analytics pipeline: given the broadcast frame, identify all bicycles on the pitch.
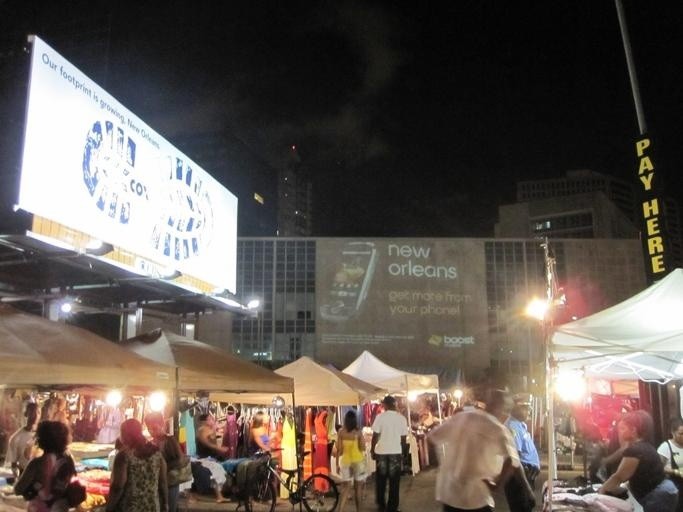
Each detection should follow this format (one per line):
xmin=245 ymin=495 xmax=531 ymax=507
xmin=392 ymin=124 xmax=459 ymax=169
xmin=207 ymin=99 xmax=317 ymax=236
xmin=243 ymin=447 xmax=339 ymax=512
